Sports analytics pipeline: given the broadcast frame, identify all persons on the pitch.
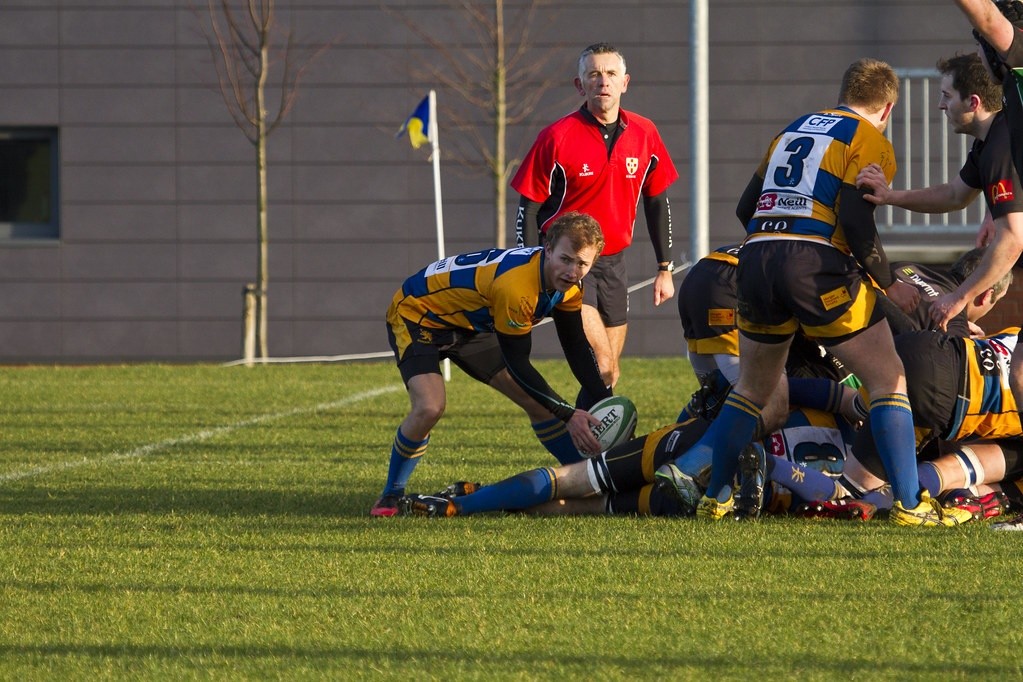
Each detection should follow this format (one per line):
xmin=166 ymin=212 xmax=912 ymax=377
xmin=399 ymin=0 xmax=1023 ymax=527
xmin=511 ymin=43 xmax=679 ymax=411
xmin=372 ymin=214 xmax=604 ymax=518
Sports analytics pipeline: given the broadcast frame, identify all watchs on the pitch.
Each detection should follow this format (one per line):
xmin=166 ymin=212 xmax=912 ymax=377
xmin=658 ymin=260 xmax=676 ymax=272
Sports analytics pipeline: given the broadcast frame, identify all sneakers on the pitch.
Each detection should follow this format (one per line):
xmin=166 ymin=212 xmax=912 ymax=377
xmin=369 ymin=488 xmax=404 ymax=518
xmin=695 ymin=491 xmax=740 ymax=521
xmin=432 ymin=481 xmax=482 ymax=500
xmin=738 ymin=441 xmax=767 ymax=520
xmin=889 ymin=489 xmax=973 ymax=529
xmin=938 ymin=491 xmax=1010 ymax=521
xmin=398 ymin=493 xmax=462 ymax=519
xmin=809 ymin=498 xmax=877 ymax=523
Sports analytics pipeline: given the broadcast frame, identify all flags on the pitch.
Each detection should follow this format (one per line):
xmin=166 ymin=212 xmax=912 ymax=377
xmin=395 ymin=96 xmax=431 ymax=150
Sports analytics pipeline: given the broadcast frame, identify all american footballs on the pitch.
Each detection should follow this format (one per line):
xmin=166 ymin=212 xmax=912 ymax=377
xmin=576 ymin=395 xmax=639 ymax=460
